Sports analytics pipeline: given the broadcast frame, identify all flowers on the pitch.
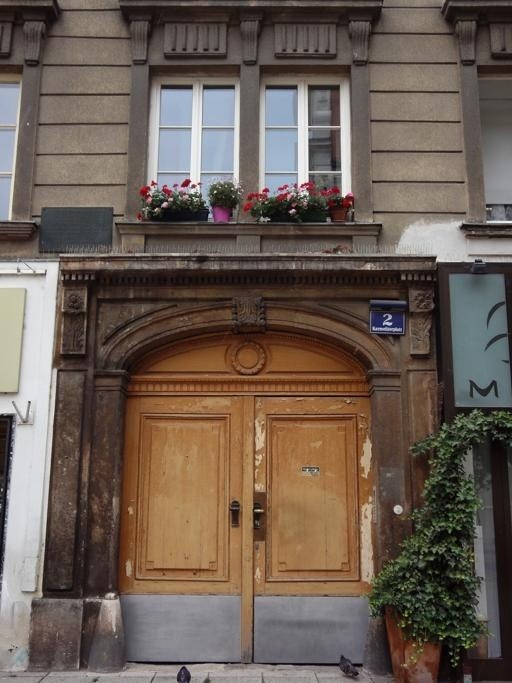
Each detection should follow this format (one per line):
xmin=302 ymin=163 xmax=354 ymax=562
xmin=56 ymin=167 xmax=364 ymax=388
xmin=134 ymin=178 xmax=202 ymax=221
xmin=245 ymin=181 xmax=354 ymax=222
xmin=207 ymin=177 xmax=244 ymax=207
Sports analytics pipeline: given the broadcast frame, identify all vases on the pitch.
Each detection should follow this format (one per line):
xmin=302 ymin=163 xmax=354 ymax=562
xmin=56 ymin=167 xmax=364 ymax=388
xmin=212 ymin=205 xmax=230 ymax=222
xmin=195 ymin=209 xmax=209 ymax=221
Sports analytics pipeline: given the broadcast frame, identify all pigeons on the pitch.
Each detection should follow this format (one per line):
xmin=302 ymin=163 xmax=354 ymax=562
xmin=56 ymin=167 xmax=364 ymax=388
xmin=177 ymin=666 xmax=191 ymax=683
xmin=339 ymin=654 xmax=359 ymax=677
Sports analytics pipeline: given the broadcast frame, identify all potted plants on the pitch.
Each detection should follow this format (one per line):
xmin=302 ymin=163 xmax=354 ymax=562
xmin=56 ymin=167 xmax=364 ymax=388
xmin=359 ymin=409 xmax=510 ymax=682
